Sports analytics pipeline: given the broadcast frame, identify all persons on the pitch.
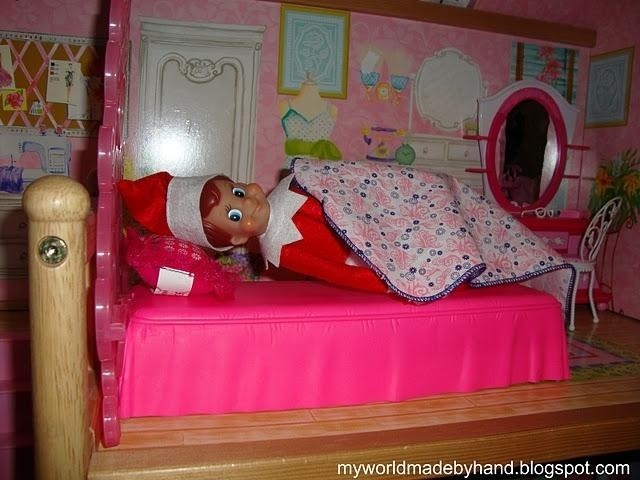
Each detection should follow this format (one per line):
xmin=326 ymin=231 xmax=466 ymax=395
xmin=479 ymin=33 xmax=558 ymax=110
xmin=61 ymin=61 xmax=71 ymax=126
xmin=115 ymin=162 xmax=399 ymax=295
xmin=276 ymin=75 xmax=339 ymax=172
